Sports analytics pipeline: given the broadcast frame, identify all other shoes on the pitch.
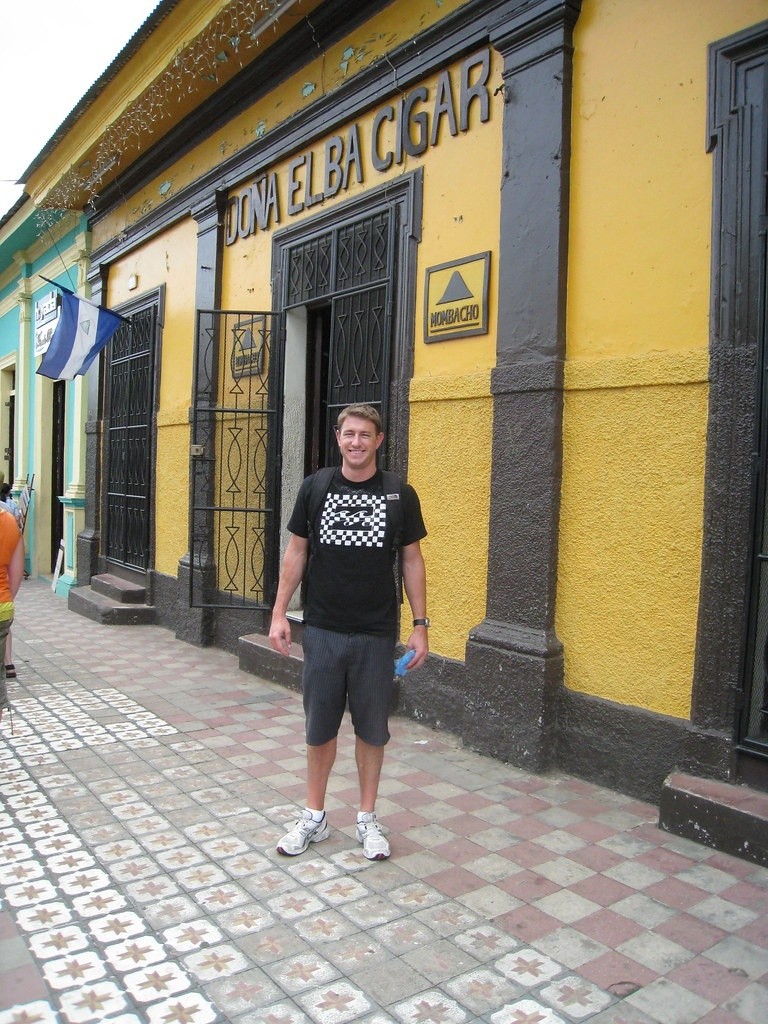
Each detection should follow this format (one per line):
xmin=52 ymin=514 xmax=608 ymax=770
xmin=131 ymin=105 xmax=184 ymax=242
xmin=5 ymin=664 xmax=16 ymax=678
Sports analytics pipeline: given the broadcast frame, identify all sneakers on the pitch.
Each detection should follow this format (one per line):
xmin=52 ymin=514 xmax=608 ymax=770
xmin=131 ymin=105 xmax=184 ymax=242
xmin=276 ymin=808 xmax=330 ymax=856
xmin=355 ymin=812 xmax=391 ymax=861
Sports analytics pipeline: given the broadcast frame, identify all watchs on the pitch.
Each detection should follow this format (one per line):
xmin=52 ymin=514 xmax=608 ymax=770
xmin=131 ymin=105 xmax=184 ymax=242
xmin=413 ymin=618 xmax=430 ymax=627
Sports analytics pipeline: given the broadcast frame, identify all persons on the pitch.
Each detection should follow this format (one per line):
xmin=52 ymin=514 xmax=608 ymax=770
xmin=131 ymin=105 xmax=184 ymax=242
xmin=0 ymin=470 xmax=25 ymax=723
xmin=269 ymin=403 xmax=429 ymax=861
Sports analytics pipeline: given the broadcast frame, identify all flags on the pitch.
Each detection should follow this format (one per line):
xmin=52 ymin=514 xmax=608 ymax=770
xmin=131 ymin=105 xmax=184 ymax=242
xmin=36 ymin=288 xmax=125 ymax=381
